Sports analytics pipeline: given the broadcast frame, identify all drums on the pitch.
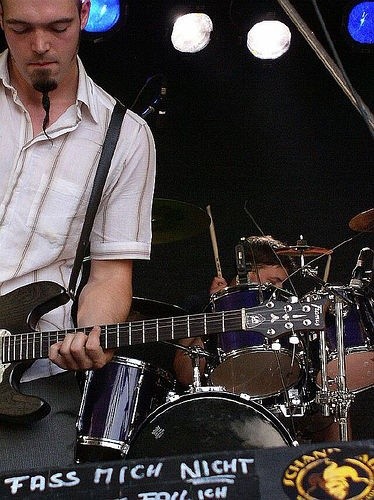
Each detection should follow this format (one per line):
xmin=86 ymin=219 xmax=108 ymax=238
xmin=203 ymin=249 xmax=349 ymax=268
xmin=74 ymin=355 xmax=176 ymax=451
xmin=300 ymin=285 xmax=374 ymax=395
xmin=198 ymin=283 xmax=305 ymax=402
xmin=122 ymin=392 xmax=298 ymax=460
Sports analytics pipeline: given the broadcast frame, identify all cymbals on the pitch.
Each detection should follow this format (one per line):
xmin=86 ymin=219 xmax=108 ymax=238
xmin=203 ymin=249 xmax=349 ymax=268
xmin=152 ymin=199 xmax=211 ymax=244
xmin=348 ymin=208 xmax=374 ymax=232
xmin=274 ymin=246 xmax=332 ymax=258
xmin=132 ymin=296 xmax=188 ymax=317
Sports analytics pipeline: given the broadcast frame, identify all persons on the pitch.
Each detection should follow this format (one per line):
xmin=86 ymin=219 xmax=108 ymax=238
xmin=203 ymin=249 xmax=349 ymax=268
xmin=0 ymin=0 xmax=157 ymax=473
xmin=173 ymin=236 xmax=294 ymax=387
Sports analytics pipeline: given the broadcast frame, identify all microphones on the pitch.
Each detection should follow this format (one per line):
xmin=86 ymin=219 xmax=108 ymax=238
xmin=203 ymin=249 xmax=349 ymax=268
xmin=158 ymin=74 xmax=168 ymax=114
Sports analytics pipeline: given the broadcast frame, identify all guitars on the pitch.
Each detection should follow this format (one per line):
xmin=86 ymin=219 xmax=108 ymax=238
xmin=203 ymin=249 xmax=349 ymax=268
xmin=0 ymin=281 xmax=328 ymax=423
